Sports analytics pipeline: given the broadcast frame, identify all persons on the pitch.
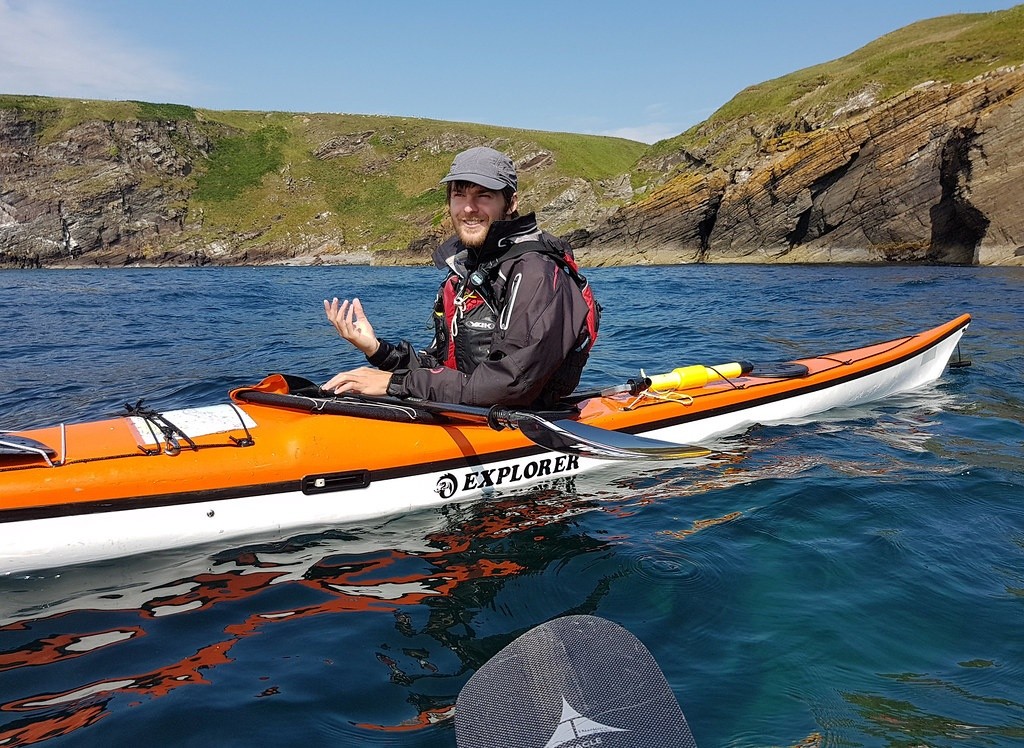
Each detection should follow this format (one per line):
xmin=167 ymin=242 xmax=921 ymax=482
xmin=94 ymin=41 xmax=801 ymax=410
xmin=321 ymin=147 xmax=591 ymax=410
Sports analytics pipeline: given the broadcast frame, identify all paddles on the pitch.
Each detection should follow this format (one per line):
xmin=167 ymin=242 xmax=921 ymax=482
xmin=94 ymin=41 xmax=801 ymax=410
xmin=454 ymin=615 xmax=698 ymax=748
xmin=317 ymin=387 xmax=722 ymax=460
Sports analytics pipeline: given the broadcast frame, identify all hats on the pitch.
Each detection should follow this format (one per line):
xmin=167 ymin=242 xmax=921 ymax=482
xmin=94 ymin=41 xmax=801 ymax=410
xmin=440 ymin=147 xmax=518 ymax=195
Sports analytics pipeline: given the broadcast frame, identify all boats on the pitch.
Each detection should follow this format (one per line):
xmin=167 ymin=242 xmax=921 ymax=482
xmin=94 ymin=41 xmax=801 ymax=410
xmin=0 ymin=312 xmax=972 ymax=578
